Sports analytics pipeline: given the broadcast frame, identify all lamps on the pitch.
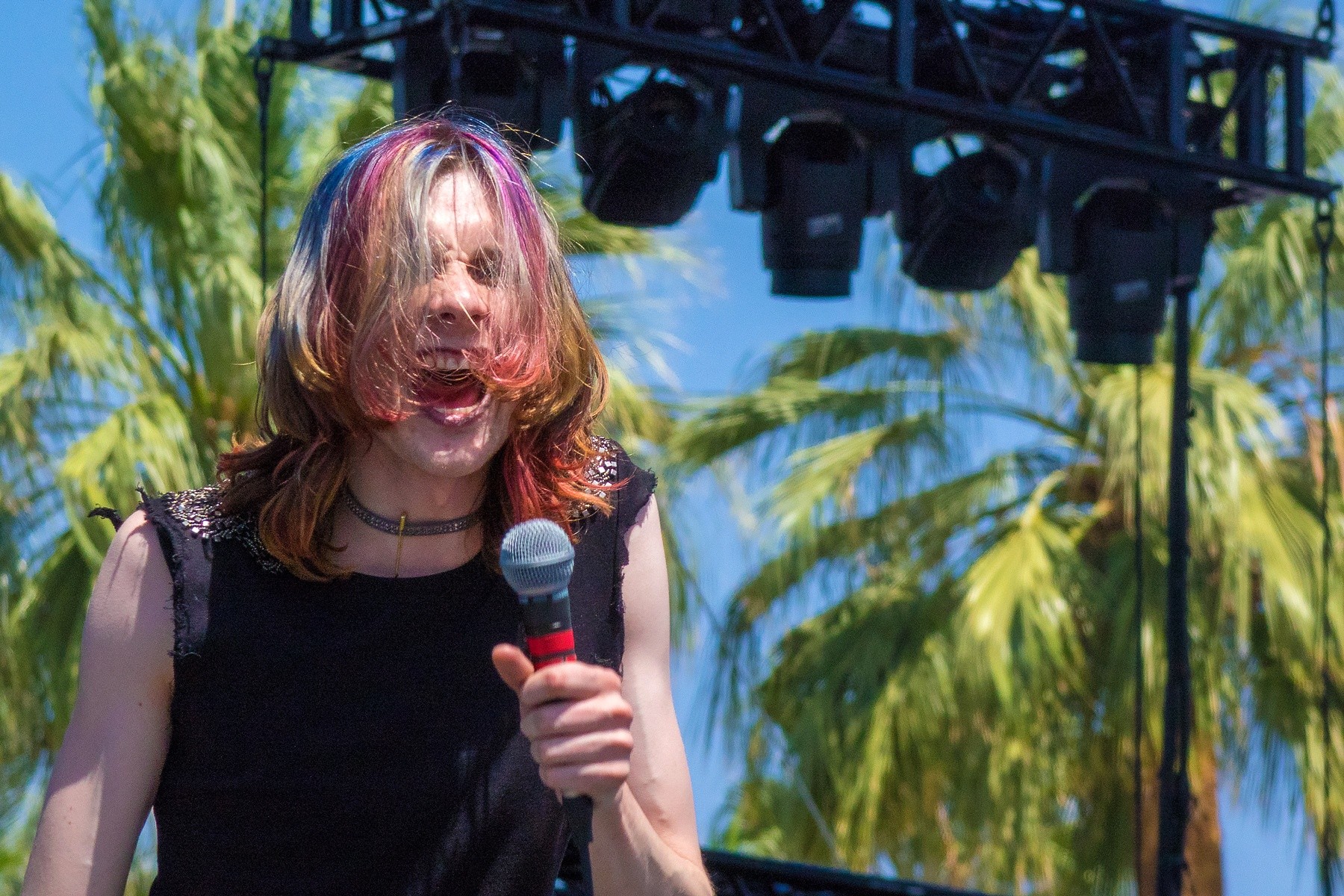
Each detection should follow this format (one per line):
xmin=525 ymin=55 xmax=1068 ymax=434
xmin=1066 ymin=178 xmax=1174 ymax=366
xmin=901 ymin=148 xmax=1040 ymax=294
xmin=577 ymin=81 xmax=723 ymax=229
xmin=399 ymin=11 xmax=566 ymax=148
xmin=763 ymin=109 xmax=875 ymax=298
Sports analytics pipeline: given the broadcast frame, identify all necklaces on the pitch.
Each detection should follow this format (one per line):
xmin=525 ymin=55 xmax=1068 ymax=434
xmin=342 ymin=485 xmax=487 ymax=578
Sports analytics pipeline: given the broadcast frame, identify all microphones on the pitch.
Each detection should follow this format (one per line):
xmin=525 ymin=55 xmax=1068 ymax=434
xmin=501 ymin=517 xmax=594 ymax=809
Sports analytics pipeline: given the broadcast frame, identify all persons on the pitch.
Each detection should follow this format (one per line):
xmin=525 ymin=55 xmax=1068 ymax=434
xmin=22 ymin=116 xmax=716 ymax=895
